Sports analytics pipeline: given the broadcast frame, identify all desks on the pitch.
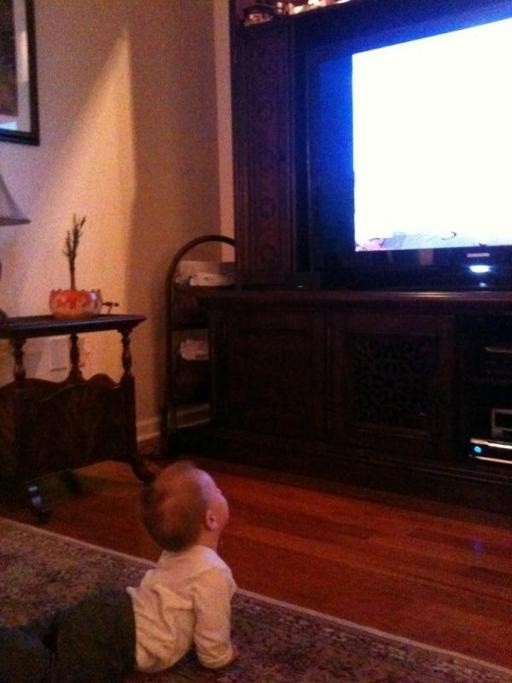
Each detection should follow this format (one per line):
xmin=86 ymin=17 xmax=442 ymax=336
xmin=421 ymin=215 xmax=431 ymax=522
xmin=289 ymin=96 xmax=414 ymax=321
xmin=1 ymin=311 xmax=156 ymax=520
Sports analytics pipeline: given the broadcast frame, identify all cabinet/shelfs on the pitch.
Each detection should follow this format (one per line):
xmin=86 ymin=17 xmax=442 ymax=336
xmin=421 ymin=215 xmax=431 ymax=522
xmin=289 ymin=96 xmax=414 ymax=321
xmin=159 ymin=229 xmax=510 ymax=512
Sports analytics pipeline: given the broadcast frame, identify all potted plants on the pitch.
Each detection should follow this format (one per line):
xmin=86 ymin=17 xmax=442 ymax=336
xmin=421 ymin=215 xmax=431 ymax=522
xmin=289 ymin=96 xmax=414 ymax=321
xmin=49 ymin=209 xmax=105 ymax=318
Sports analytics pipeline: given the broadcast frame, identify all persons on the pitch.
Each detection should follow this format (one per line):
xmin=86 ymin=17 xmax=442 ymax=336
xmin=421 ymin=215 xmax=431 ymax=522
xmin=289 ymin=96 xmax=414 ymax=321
xmin=0 ymin=462 xmax=242 ymax=682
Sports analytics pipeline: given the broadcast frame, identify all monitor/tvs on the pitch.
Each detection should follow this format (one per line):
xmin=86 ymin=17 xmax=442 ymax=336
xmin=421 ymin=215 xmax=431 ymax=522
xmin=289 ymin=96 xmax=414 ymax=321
xmin=351 ymin=16 xmax=512 ymax=252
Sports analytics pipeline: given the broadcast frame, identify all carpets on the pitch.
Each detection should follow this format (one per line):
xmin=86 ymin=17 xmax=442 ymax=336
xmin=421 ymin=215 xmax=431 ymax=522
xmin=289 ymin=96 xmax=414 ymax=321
xmin=1 ymin=515 xmax=510 ymax=683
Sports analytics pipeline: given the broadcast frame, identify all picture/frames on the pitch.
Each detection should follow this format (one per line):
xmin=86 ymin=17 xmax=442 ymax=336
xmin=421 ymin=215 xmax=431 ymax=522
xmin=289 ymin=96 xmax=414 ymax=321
xmin=0 ymin=0 xmax=43 ymax=147
